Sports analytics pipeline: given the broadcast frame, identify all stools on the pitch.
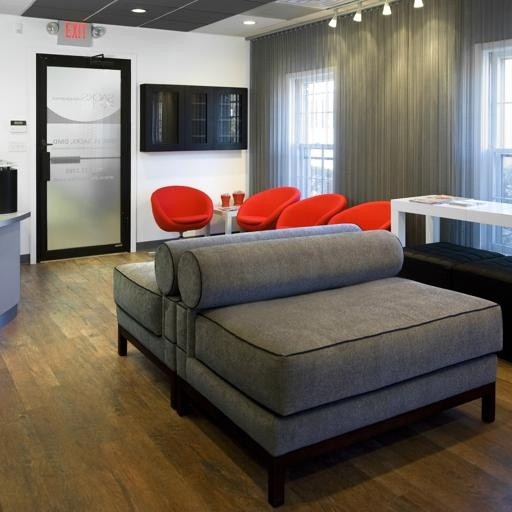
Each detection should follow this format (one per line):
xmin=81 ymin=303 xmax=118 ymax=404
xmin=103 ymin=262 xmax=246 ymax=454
xmin=447 ymin=256 xmax=511 ymax=363
xmin=397 ymin=242 xmax=505 ymax=288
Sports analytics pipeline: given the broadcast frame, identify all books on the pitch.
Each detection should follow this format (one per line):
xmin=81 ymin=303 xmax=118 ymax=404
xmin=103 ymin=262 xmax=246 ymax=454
xmin=453 ymin=198 xmax=488 ymax=208
xmin=409 ymin=192 xmax=461 ymax=204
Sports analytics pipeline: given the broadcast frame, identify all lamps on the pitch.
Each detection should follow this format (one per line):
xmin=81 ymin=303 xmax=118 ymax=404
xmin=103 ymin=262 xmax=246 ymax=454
xmin=325 ymin=0 xmax=425 ymax=29
xmin=46 ymin=19 xmax=106 ymax=47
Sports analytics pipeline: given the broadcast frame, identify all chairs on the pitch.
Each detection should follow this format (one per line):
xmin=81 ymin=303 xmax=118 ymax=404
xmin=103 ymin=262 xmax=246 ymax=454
xmin=328 ymin=200 xmax=391 ymax=231
xmin=113 ymin=223 xmax=362 ymax=408
xmin=176 ymin=230 xmax=503 ymax=507
xmin=237 ymin=187 xmax=301 ymax=229
xmin=151 ymin=186 xmax=214 ymax=238
xmin=274 ymin=193 xmax=348 ymax=229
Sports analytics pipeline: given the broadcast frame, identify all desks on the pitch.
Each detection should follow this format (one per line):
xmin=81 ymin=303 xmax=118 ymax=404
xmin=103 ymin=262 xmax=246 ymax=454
xmin=390 ymin=194 xmax=512 ymax=248
